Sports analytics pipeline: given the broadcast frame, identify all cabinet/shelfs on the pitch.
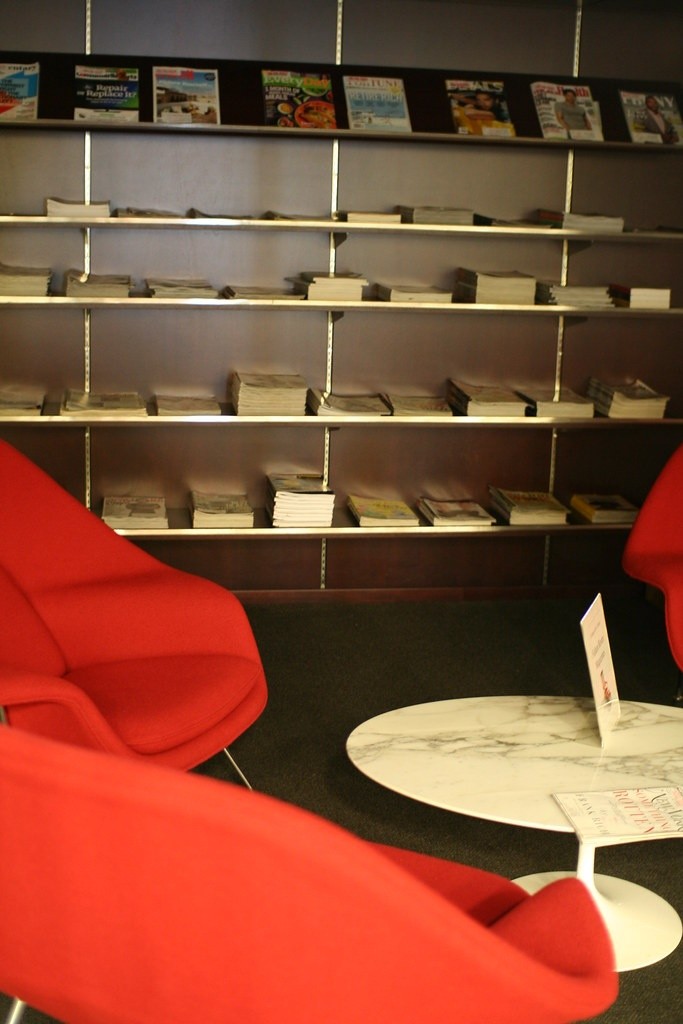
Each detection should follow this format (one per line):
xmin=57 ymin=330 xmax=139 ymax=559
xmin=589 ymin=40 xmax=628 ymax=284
xmin=0 ymin=49 xmax=683 ymax=603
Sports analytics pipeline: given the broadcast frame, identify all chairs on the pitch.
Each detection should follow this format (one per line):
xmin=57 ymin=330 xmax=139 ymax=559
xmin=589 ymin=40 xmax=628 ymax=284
xmin=0 ymin=438 xmax=268 ymax=794
xmin=0 ymin=724 xmax=617 ymax=1024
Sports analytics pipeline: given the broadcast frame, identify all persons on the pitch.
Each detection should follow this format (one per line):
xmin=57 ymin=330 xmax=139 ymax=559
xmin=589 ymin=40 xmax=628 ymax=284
xmin=644 ymin=96 xmax=679 ymax=143
xmin=447 ymin=92 xmax=504 ymax=122
xmin=555 ymin=88 xmax=592 ymax=139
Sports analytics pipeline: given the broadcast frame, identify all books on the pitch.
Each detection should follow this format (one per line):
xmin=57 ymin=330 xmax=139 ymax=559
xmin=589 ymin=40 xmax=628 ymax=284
xmin=153 ymin=66 xmax=221 ymax=124
xmin=0 ymin=373 xmax=669 ymax=418
xmin=446 ymin=80 xmax=516 ymax=136
xmin=262 ymin=70 xmax=337 ymax=129
xmin=46 ymin=196 xmax=683 ymax=234
xmin=0 ymin=63 xmax=39 ymax=120
xmin=74 ymin=66 xmax=139 ymax=122
xmin=101 ymin=473 xmax=640 ymax=529
xmin=551 ymin=785 xmax=683 ymax=849
xmin=343 ymin=76 xmax=412 ymax=131
xmin=618 ymin=88 xmax=683 ymax=144
xmin=530 ymin=82 xmax=604 ymax=140
xmin=0 ymin=264 xmax=671 ymax=308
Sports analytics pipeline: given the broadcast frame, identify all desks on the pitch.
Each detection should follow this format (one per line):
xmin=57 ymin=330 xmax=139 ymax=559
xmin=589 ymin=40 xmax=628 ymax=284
xmin=346 ymin=695 xmax=683 ymax=973
